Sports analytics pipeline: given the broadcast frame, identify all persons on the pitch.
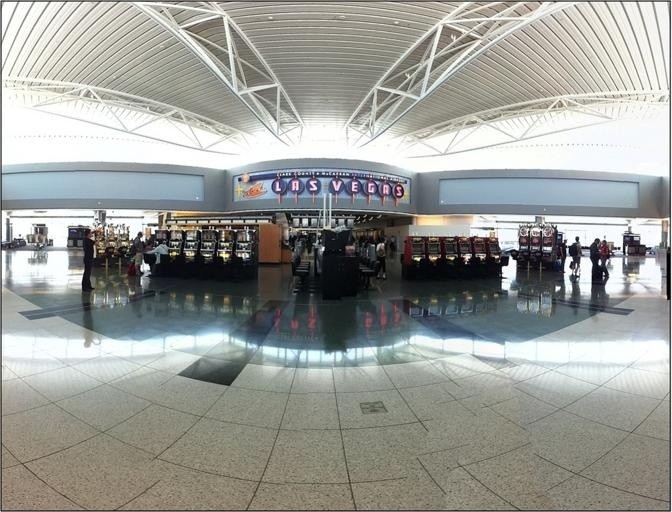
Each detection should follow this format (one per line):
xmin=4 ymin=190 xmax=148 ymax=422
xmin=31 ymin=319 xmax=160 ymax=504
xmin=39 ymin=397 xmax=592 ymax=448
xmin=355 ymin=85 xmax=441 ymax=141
xmin=375 ymin=237 xmax=388 ymax=280
xmin=601 ymin=239 xmax=611 ymax=277
xmin=134 ymin=232 xmax=143 ymax=276
xmin=590 ymin=238 xmax=601 ymax=269
xmin=145 ymin=242 xmax=154 ymax=251
xmin=559 ymin=238 xmax=568 ymax=272
xmin=81 ymin=229 xmax=100 ymax=293
xmin=571 ymin=237 xmax=583 ymax=278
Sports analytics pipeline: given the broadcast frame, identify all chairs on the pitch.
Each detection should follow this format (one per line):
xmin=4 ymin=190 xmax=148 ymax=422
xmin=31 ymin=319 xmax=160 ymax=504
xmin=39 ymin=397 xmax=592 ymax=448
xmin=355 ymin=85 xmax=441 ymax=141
xmin=291 ymin=256 xmax=315 ymax=297
xmin=360 ymin=255 xmax=381 ymax=292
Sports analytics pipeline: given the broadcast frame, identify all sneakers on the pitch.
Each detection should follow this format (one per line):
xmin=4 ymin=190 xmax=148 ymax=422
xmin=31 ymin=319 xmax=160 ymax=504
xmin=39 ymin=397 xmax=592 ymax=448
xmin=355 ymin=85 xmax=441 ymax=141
xmin=83 ymin=287 xmax=95 ymax=291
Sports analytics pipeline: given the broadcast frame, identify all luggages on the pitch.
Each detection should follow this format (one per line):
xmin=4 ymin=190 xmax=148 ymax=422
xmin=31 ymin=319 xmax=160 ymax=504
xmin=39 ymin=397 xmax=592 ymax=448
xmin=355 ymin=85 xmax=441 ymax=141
xmin=592 ymin=267 xmax=602 ymax=280
xmin=127 ymin=258 xmax=135 ymax=275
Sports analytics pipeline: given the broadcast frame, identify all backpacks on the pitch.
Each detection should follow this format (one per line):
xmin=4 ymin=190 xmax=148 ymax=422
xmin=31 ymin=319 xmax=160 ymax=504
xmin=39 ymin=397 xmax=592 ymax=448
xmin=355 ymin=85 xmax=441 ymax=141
xmin=569 ymin=245 xmax=577 ymax=256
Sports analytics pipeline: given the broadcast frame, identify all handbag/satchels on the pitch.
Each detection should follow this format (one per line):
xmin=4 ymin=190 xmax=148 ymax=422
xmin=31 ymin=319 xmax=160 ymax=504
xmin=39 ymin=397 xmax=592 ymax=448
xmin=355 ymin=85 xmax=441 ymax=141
xmin=607 ymin=254 xmax=610 ymax=259
xmin=379 ymin=247 xmax=384 ymax=256
xmin=569 ymin=262 xmax=575 ymax=269
xmin=131 ymin=245 xmax=136 ymax=255
